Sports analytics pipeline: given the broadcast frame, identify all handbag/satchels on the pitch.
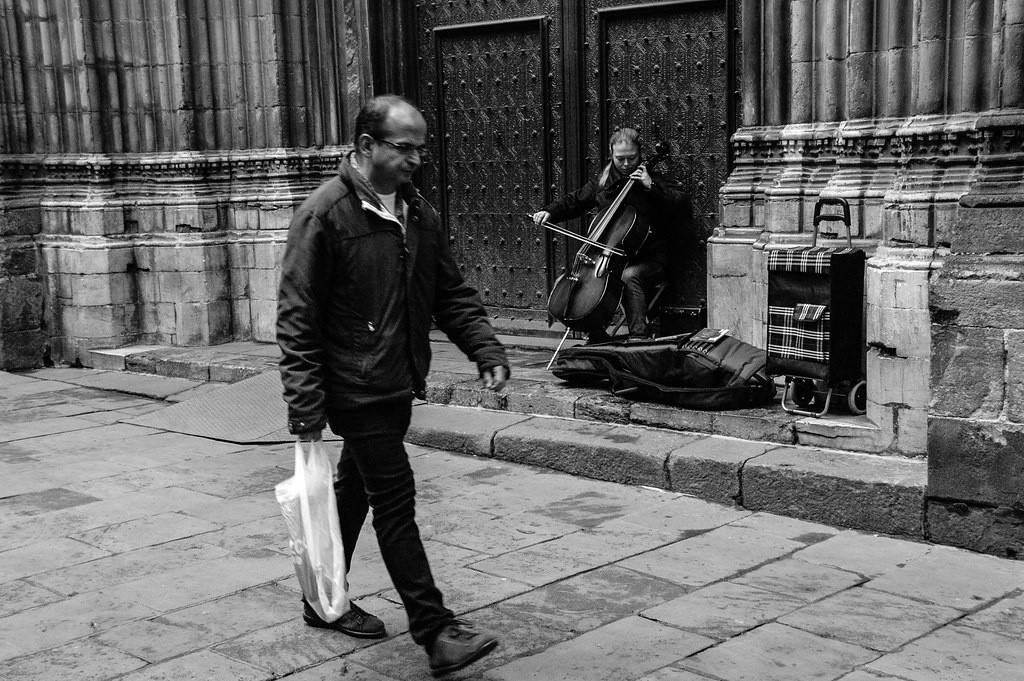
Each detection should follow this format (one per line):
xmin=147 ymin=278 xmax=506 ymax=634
xmin=275 ymin=436 xmax=351 ymax=623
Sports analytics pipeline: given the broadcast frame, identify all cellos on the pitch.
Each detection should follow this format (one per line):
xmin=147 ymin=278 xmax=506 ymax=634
xmin=543 ymin=150 xmax=653 ymax=373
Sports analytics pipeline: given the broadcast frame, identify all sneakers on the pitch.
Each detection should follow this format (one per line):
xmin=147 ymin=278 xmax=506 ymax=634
xmin=429 ymin=619 xmax=500 ymax=678
xmin=303 ymin=601 xmax=387 ymax=638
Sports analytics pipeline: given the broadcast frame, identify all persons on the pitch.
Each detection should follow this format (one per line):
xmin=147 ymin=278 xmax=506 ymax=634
xmin=275 ymin=97 xmax=510 ymax=677
xmin=533 ymin=128 xmax=688 ymax=346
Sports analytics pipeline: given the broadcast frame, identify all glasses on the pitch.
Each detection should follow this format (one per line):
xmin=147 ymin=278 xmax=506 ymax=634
xmin=374 ymin=138 xmax=427 ymax=156
